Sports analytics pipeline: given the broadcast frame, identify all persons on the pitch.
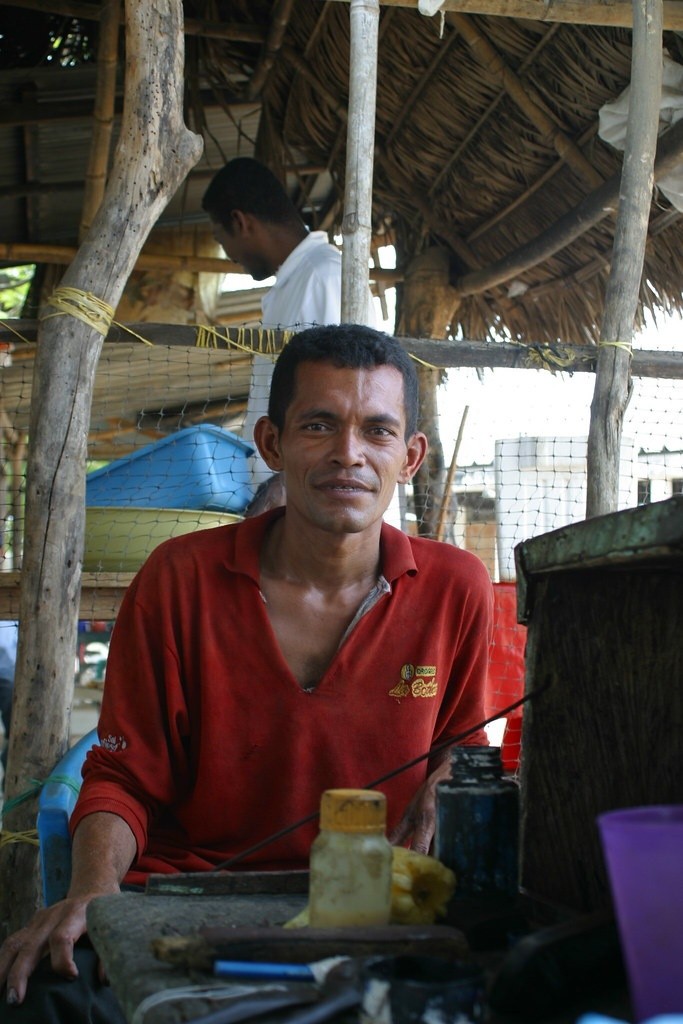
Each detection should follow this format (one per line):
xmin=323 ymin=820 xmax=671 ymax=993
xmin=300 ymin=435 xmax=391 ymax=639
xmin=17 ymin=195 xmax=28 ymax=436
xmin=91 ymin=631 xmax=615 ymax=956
xmin=1 ymin=323 xmax=494 ymax=1024
xmin=201 ymin=157 xmax=402 ymax=532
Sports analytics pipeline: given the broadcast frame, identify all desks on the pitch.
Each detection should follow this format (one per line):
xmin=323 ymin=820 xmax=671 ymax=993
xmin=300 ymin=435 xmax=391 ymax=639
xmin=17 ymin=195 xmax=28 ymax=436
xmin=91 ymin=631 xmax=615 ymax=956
xmin=83 ymin=885 xmax=308 ymax=1023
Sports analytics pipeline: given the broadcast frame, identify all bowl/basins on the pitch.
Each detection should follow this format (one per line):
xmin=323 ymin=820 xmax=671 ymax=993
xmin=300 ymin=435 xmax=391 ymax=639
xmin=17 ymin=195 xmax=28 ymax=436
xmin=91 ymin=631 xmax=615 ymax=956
xmin=81 ymin=422 xmax=257 ymax=574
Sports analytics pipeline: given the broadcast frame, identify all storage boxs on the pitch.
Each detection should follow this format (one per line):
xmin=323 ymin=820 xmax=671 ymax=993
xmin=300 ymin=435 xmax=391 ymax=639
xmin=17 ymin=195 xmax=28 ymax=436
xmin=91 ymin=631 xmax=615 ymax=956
xmin=86 ymin=424 xmax=255 ymax=513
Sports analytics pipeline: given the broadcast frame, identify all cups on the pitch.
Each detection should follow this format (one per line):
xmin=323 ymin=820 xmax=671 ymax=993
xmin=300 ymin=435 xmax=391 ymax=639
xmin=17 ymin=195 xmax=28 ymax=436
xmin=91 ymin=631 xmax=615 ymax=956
xmin=600 ymin=805 xmax=683 ymax=1023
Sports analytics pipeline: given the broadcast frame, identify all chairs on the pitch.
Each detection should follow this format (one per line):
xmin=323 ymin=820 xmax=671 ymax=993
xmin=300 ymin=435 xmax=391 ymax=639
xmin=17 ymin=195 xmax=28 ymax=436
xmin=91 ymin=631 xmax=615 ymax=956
xmin=35 ymin=727 xmax=103 ymax=910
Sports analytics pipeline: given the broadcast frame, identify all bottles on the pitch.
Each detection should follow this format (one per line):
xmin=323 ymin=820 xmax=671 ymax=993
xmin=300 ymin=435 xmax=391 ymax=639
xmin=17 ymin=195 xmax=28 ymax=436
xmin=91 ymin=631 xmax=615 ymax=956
xmin=309 ymin=789 xmax=392 ymax=928
xmin=437 ymin=744 xmax=521 ymax=943
